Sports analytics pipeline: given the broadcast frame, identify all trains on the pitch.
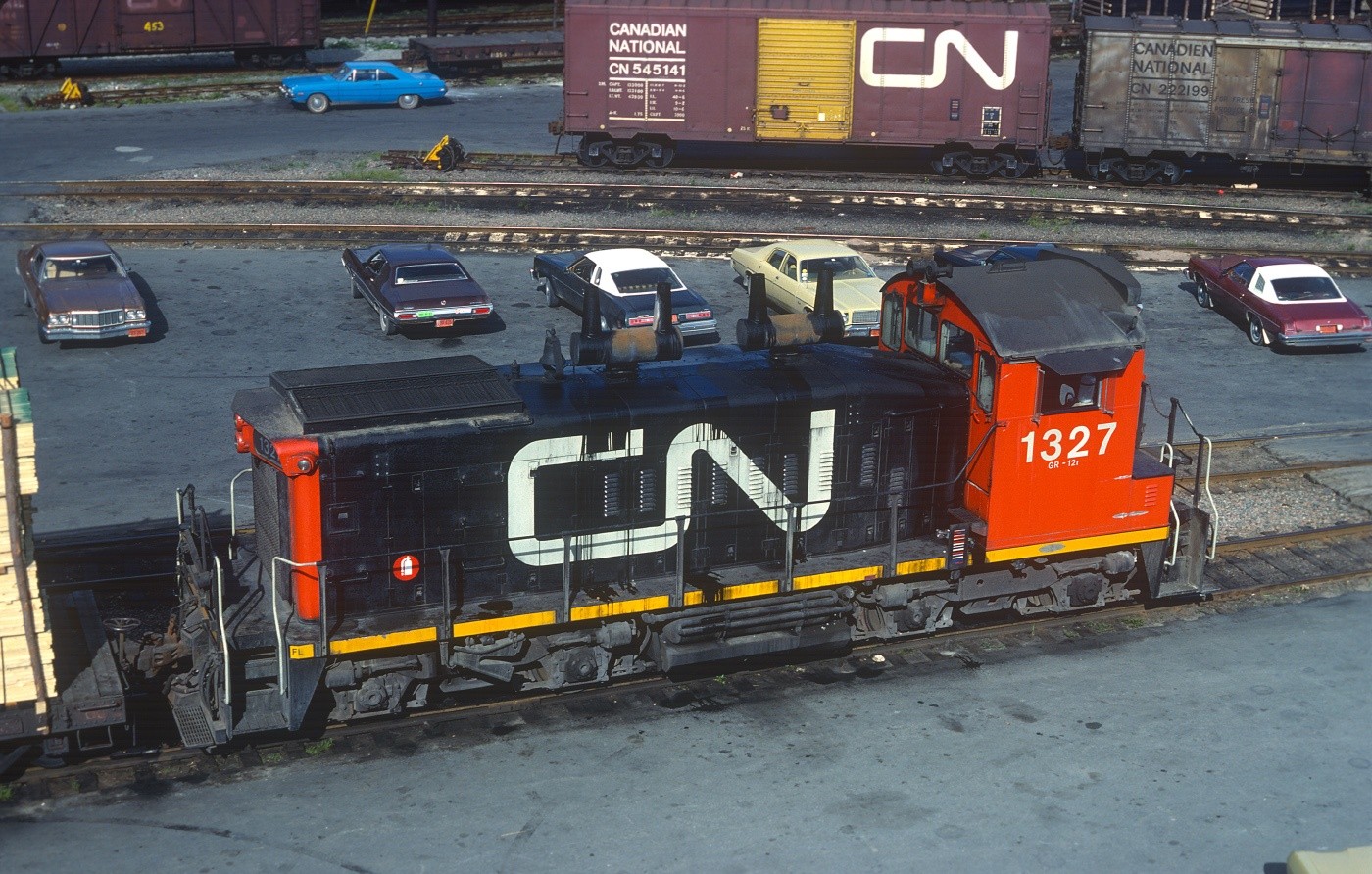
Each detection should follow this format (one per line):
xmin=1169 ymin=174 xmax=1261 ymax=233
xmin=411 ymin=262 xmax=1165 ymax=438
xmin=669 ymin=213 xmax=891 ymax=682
xmin=0 ymin=0 xmax=566 ymax=84
xmin=0 ymin=240 xmax=1220 ymax=786
xmin=544 ymin=0 xmax=1372 ymax=198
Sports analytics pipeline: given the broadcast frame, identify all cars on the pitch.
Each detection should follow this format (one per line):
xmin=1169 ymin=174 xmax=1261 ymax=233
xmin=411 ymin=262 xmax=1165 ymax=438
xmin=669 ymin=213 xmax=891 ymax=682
xmin=340 ymin=241 xmax=494 ymax=337
xmin=531 ymin=247 xmax=718 ymax=338
xmin=1183 ymin=253 xmax=1372 ymax=348
xmin=730 ymin=238 xmax=888 ymax=339
xmin=276 ymin=60 xmax=448 ymax=114
xmin=14 ymin=235 xmax=152 ymax=344
